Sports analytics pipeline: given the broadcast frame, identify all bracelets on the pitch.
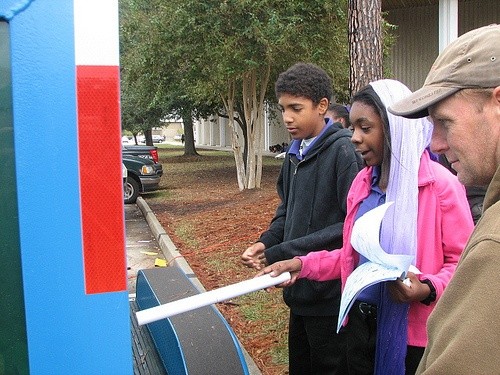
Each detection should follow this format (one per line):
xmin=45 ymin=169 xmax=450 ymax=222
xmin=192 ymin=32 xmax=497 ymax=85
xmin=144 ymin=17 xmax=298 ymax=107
xmin=421 ymin=278 xmax=436 ymax=306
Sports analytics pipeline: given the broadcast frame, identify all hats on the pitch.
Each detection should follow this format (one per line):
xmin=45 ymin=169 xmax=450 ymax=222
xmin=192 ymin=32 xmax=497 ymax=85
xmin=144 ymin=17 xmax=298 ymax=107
xmin=388 ymin=23 xmax=500 ymax=119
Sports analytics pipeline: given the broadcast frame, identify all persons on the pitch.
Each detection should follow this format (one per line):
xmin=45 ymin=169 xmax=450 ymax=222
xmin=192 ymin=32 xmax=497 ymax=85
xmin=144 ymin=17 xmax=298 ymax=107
xmin=240 ymin=63 xmax=366 ymax=375
xmin=387 ymin=23 xmax=500 ymax=374
xmin=255 ymin=78 xmax=474 ymax=375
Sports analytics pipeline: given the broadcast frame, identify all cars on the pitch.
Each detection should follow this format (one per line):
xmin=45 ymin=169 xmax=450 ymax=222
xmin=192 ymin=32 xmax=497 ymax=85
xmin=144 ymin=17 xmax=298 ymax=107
xmin=120 ymin=135 xmax=164 ymax=145
xmin=122 ymin=145 xmax=163 ymax=204
